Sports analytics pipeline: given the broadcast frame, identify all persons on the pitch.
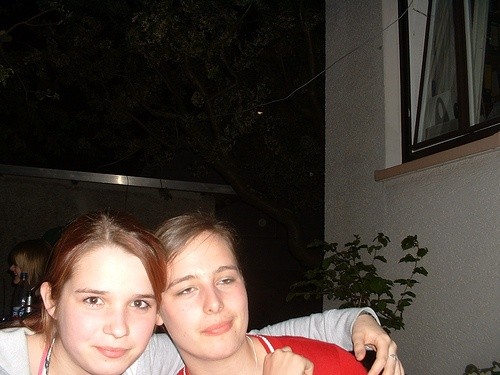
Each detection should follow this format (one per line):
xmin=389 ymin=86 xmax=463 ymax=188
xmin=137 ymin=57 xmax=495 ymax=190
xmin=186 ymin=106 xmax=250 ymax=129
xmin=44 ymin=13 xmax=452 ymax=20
xmin=11 ymin=240 xmax=50 ymax=317
xmin=1 ymin=209 xmax=407 ymax=375
xmin=149 ymin=213 xmax=368 ymax=375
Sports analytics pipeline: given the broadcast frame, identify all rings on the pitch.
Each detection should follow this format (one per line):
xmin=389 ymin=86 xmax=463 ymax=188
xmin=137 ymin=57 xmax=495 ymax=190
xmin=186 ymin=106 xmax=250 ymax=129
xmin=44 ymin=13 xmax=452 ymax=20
xmin=388 ymin=353 xmax=399 ymax=361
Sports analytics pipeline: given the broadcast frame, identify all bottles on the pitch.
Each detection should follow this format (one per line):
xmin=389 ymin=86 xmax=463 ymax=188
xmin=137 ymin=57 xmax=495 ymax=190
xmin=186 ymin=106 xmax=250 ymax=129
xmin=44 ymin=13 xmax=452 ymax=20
xmin=12 ymin=273 xmax=30 ymax=319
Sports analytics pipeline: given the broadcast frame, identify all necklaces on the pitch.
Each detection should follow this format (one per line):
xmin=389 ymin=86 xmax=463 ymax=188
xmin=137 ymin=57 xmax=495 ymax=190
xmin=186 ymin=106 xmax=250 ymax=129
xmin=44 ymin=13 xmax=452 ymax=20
xmin=45 ymin=334 xmax=57 ymax=375
xmin=183 ymin=335 xmax=258 ymax=375
